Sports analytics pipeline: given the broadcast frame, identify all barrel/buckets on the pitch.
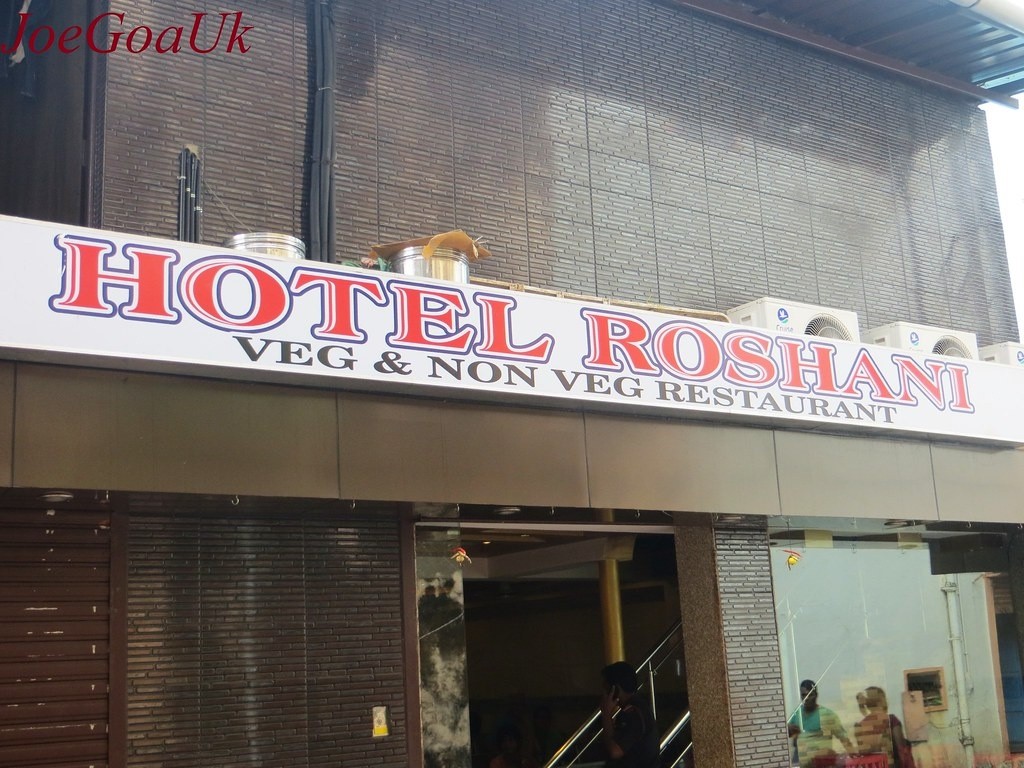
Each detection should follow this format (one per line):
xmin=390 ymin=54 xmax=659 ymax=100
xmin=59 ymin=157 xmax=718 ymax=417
xmin=391 ymin=245 xmax=471 ymax=285
xmin=227 ymin=232 xmax=307 ymax=261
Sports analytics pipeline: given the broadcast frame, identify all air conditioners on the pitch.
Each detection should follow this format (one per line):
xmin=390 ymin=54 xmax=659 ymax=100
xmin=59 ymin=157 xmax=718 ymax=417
xmin=977 ymin=341 xmax=1024 ymax=367
xmin=725 ymin=296 xmax=862 ymax=341
xmin=862 ymin=322 xmax=981 ymax=361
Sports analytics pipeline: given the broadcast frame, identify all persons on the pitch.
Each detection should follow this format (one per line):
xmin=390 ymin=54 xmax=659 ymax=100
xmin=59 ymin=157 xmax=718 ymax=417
xmin=599 ymin=662 xmax=659 ymax=768
xmin=786 ymin=680 xmax=854 ymax=768
xmin=855 ymin=686 xmax=915 ymax=768
